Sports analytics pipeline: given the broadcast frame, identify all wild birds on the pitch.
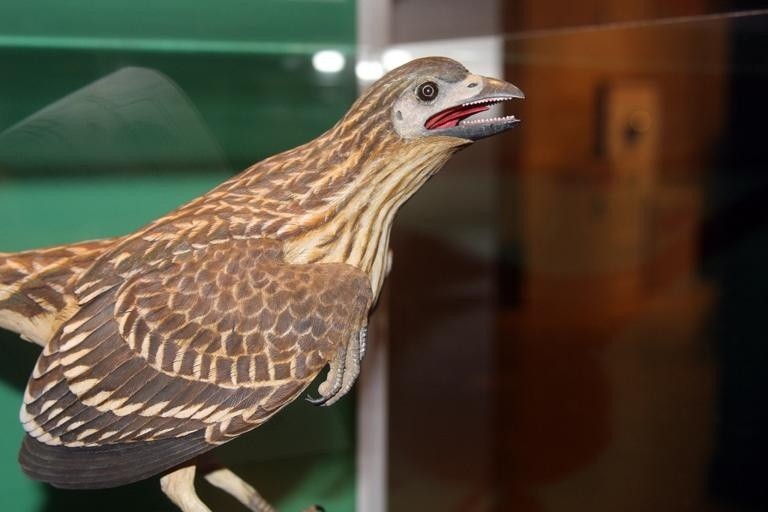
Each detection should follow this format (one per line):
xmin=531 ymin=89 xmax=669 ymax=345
xmin=1 ymin=56 xmax=525 ymax=511
